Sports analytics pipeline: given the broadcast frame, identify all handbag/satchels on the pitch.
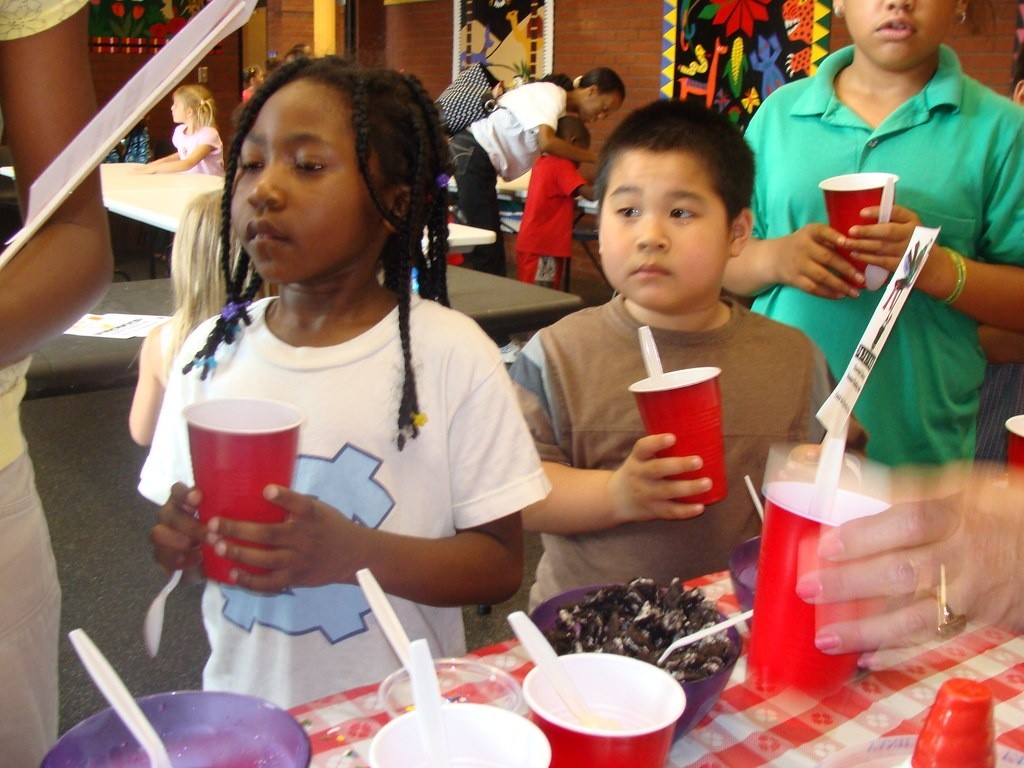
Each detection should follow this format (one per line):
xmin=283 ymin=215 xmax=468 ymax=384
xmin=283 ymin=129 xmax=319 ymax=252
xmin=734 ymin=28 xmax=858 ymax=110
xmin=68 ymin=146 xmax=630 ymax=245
xmin=434 ymin=62 xmax=508 ymax=136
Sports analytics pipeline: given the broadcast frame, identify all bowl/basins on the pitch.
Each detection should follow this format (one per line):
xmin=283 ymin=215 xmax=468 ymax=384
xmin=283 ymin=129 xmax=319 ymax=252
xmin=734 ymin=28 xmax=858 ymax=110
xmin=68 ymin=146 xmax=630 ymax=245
xmin=39 ymin=689 xmax=311 ymax=768
xmin=529 ymin=584 xmax=742 ymax=743
xmin=725 ymin=534 xmax=761 ymax=628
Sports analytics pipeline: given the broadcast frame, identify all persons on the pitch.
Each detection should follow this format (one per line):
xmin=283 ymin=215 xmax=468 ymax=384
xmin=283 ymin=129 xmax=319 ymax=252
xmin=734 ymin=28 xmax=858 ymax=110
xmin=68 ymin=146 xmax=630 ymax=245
xmin=242 ymin=45 xmax=312 ymax=102
xmin=974 ymin=60 xmax=1024 ymax=464
xmin=508 ymin=100 xmax=868 ymax=617
xmin=131 ymin=189 xmax=240 ymax=446
xmin=448 ymin=68 xmax=626 ymax=275
xmin=1 ymin=0 xmax=117 ymax=768
xmin=723 ymin=0 xmax=1024 ymax=468
xmin=515 ymin=117 xmax=596 ymax=290
xmin=137 ymin=56 xmax=553 ymax=709
xmin=128 ymin=85 xmax=224 ymax=175
xmin=796 ymin=478 xmax=1024 ymax=668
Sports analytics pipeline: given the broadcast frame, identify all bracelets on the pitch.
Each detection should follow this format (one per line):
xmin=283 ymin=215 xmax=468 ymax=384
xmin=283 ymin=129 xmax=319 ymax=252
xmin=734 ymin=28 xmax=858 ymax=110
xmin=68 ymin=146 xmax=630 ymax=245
xmin=925 ymin=246 xmax=968 ymax=306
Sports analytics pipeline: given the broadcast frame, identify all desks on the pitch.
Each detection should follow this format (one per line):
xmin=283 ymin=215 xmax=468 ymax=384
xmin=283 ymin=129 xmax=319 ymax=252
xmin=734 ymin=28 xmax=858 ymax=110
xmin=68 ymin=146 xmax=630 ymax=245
xmin=93 ymin=474 xmax=1024 ymax=768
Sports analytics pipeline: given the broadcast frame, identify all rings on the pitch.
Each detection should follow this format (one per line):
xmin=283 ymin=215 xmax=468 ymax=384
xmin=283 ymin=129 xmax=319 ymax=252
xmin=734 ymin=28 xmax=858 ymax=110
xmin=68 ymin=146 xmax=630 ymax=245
xmin=936 ymin=594 xmax=966 ymax=637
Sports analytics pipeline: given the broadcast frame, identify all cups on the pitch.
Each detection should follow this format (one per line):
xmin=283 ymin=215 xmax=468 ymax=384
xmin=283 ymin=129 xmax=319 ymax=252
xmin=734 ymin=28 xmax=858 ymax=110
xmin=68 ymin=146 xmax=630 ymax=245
xmin=374 ymin=656 xmax=524 ymax=719
xmin=1004 ymin=414 xmax=1024 ymax=473
xmin=181 ymin=396 xmax=304 ymax=586
xmin=747 ymin=479 xmax=895 ymax=700
xmin=522 ymin=653 xmax=686 ymax=768
xmin=911 ymin=677 xmax=997 ymax=768
xmin=368 ymin=700 xmax=553 ymax=768
xmin=628 ymin=366 xmax=728 ymax=507
xmin=819 ymin=172 xmax=900 ymax=289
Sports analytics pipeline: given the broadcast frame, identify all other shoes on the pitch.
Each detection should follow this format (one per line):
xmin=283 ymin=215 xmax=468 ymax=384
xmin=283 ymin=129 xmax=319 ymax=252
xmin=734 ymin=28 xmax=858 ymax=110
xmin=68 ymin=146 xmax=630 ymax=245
xmin=500 ymin=341 xmax=522 ymax=364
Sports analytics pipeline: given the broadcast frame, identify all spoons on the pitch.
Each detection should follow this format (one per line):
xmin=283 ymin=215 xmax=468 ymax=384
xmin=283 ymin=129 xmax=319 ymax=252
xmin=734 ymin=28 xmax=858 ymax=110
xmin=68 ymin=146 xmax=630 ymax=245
xmin=866 ymin=175 xmax=895 ymax=292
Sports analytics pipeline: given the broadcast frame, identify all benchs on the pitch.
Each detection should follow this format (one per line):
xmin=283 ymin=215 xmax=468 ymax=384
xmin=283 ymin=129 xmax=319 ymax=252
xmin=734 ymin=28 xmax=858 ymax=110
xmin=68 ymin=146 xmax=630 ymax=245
xmin=430 ymin=265 xmax=580 ymax=350
xmin=100 ymin=161 xmax=497 ymax=264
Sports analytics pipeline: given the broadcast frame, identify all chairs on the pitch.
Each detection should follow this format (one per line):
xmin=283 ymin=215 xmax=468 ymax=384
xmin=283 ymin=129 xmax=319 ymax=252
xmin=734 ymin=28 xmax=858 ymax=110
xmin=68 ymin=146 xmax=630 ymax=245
xmin=560 ymin=206 xmax=613 ymax=292
